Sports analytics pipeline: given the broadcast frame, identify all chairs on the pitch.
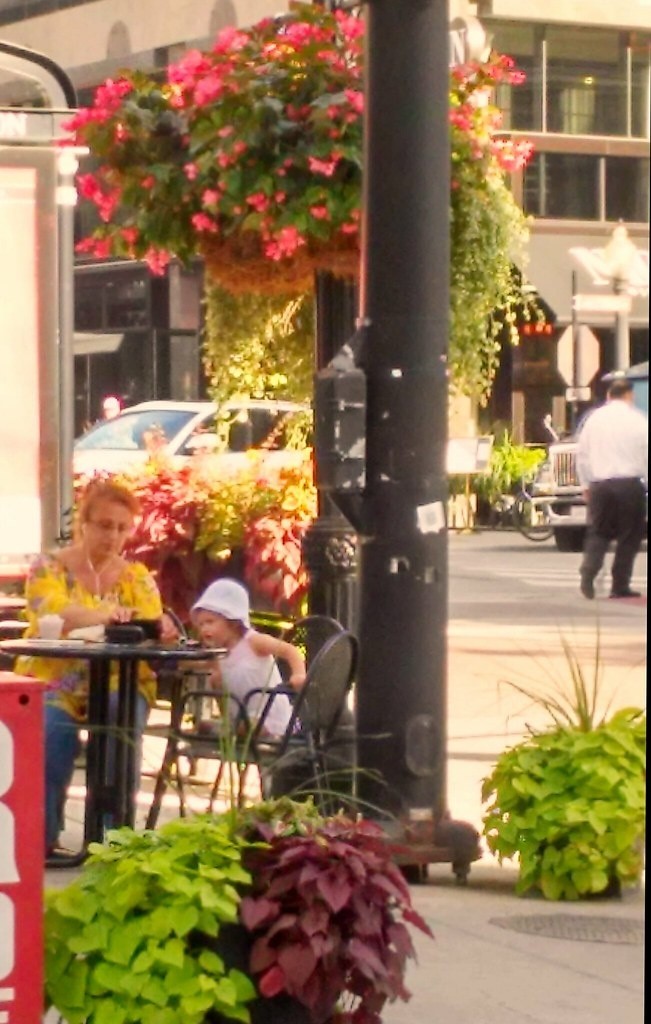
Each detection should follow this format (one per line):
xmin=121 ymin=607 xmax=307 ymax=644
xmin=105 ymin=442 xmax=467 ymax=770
xmin=173 ymin=613 xmax=345 ymax=818
xmin=145 ymin=630 xmax=360 ymax=839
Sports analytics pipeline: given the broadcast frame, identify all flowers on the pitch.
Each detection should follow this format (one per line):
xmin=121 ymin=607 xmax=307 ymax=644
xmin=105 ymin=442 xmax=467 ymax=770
xmin=54 ymin=0 xmax=532 ymax=279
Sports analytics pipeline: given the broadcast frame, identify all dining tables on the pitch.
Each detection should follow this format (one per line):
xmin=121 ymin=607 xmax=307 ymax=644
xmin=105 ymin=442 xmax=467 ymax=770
xmin=2 ymin=639 xmax=230 ymax=869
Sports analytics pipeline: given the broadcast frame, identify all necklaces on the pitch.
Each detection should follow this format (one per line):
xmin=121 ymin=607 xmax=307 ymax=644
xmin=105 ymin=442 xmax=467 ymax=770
xmin=85 ymin=550 xmax=112 ymax=598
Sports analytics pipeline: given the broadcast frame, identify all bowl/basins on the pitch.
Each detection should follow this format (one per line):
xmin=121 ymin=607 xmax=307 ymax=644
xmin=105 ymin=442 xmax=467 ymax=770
xmin=38 ymin=616 xmax=64 ymax=641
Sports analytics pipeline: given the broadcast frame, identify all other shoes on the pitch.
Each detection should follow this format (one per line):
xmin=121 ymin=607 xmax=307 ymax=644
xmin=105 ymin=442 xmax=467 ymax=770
xmin=580 ymin=568 xmax=595 ymax=599
xmin=610 ymin=584 xmax=640 ymax=598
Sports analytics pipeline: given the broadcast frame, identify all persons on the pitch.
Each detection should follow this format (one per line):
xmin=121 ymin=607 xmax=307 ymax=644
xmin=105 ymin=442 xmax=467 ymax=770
xmin=577 ymin=381 xmax=651 ymax=600
xmin=16 ymin=480 xmax=162 ymax=858
xmin=126 ymin=412 xmax=166 ymax=450
xmin=188 ymin=578 xmax=306 ymax=743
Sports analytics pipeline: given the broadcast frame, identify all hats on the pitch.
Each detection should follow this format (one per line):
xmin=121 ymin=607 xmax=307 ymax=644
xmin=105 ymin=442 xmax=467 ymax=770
xmin=187 ymin=577 xmax=256 ymax=635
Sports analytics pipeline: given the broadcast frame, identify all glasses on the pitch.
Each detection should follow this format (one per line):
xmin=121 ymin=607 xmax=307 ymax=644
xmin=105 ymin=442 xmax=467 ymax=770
xmin=86 ymin=516 xmax=131 ymax=532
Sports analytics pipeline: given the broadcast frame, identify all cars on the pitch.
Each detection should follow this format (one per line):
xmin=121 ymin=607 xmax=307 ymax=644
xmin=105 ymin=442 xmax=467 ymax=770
xmin=73 ymin=403 xmax=316 ymax=492
xmin=528 ymin=362 xmax=650 ymax=549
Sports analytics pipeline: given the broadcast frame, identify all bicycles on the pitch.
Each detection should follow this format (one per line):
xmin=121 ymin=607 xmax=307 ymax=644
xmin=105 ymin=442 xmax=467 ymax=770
xmin=510 ymin=415 xmax=557 ymax=542
